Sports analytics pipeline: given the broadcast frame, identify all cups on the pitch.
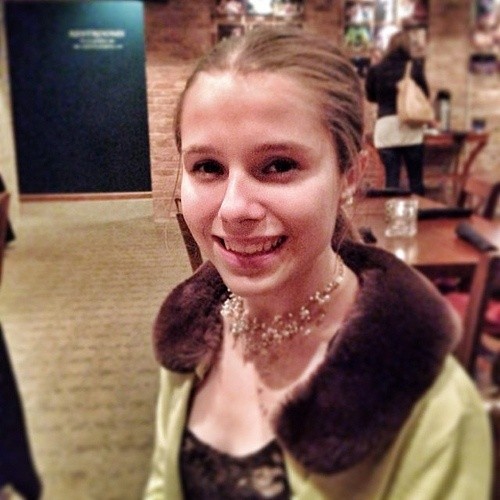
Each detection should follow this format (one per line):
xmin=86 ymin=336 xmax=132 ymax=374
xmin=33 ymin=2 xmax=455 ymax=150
xmin=387 ymin=198 xmax=419 ymax=237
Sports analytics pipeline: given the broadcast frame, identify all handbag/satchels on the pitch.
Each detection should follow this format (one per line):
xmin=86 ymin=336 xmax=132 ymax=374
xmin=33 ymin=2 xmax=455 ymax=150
xmin=398 ymin=62 xmax=435 ymax=128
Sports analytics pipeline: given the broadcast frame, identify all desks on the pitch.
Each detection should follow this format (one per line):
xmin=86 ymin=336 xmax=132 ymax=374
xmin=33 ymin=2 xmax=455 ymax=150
xmin=344 ymin=195 xmax=500 ymax=296
xmin=426 ymin=132 xmax=457 ymax=145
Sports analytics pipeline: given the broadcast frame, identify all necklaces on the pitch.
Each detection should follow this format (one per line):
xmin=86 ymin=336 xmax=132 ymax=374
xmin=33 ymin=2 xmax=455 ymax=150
xmin=222 ymin=253 xmax=344 ymax=377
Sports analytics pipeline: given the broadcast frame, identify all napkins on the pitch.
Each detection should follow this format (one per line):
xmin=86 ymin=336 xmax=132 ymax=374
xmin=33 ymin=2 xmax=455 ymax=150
xmin=357 ymin=226 xmax=378 ymax=243
xmin=413 ymin=206 xmax=473 ymax=221
xmin=362 ymin=187 xmax=409 ymax=199
xmin=455 ymin=222 xmax=496 ymax=252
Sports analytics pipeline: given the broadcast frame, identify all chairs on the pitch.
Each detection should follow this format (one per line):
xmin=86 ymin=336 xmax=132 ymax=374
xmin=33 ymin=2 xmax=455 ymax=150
xmin=172 ymin=195 xmax=202 ymax=276
xmin=441 ymin=118 xmax=500 ymax=442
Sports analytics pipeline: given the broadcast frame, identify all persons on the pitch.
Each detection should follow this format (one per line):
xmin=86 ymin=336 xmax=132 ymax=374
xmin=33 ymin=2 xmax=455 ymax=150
xmin=1 ymin=178 xmax=42 ymax=499
xmin=363 ymin=32 xmax=430 ymax=196
xmin=143 ymin=23 xmax=492 ymax=500
